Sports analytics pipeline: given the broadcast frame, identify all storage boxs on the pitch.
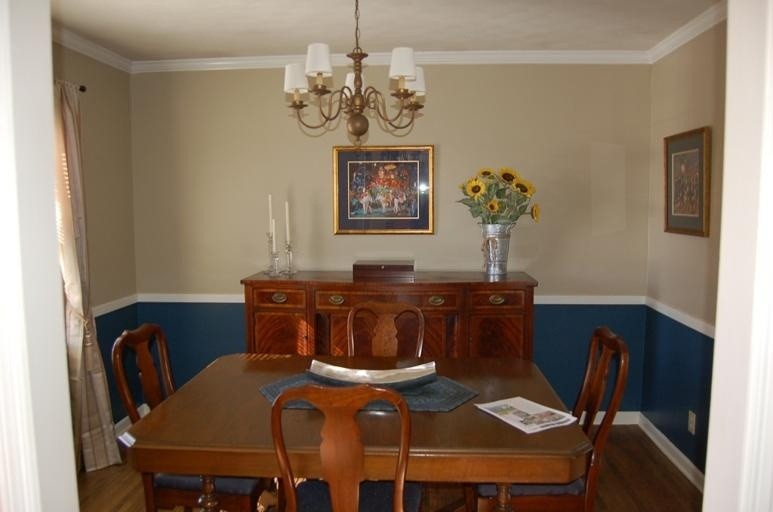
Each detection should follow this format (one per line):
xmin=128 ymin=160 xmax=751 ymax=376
xmin=353 ymin=259 xmax=415 ymax=281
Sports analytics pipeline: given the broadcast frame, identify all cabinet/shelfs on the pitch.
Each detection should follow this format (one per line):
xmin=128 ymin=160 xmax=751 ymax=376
xmin=242 ymin=281 xmax=312 ymax=355
xmin=464 ymin=281 xmax=537 ymax=359
xmin=313 ymin=281 xmax=464 ymax=361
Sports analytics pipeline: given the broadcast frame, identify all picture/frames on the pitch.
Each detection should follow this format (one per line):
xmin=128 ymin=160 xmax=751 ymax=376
xmin=662 ymin=126 xmax=713 ymax=237
xmin=332 ymin=144 xmax=435 ymax=235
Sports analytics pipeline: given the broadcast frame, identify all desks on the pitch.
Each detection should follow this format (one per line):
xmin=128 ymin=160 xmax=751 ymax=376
xmin=116 ymin=352 xmax=595 ymax=512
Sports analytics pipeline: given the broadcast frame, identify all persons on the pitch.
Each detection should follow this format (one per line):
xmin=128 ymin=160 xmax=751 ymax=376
xmin=357 ymin=187 xmax=408 ymax=216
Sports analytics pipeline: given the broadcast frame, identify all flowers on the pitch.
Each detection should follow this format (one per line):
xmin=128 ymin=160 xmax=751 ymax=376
xmin=455 ymin=167 xmax=541 ymax=224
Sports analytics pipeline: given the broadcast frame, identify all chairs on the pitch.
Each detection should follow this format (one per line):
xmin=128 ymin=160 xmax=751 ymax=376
xmin=270 ymin=383 xmax=423 ymax=512
xmin=468 ymin=326 xmax=630 ymax=512
xmin=347 ymin=300 xmax=425 ymax=357
xmin=110 ymin=321 xmax=265 ymax=512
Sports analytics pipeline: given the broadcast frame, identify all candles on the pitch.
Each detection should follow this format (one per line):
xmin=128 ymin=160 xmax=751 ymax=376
xmin=271 ymin=219 xmax=276 ymax=254
xmin=268 ymin=194 xmax=272 ymax=237
xmin=285 ymin=201 xmax=289 ymax=244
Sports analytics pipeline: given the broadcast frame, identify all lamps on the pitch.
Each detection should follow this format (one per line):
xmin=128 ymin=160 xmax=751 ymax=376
xmin=283 ymin=0 xmax=426 ymax=148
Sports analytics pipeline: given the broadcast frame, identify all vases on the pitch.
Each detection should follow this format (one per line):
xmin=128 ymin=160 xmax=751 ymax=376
xmin=479 ymin=222 xmax=516 ymax=276
